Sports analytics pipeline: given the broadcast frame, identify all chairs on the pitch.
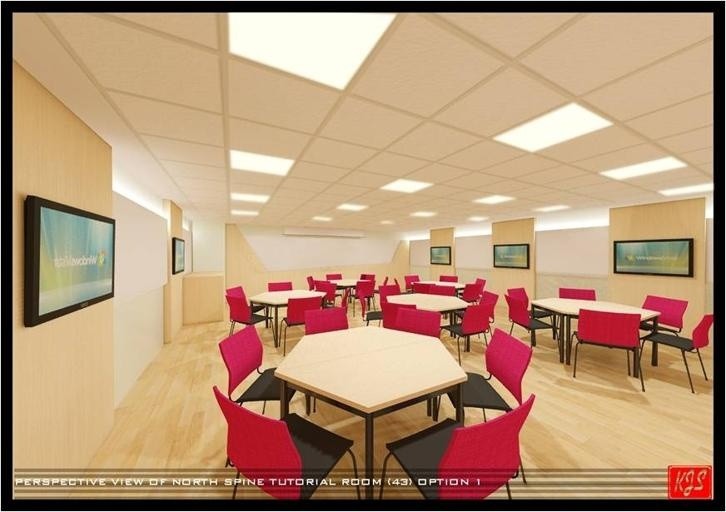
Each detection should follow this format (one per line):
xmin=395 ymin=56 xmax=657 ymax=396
xmin=268 ymin=282 xmax=292 ymax=328
xmin=455 ymin=291 xmax=498 ymax=339
xmin=279 ymin=296 xmax=321 ymax=356
xmin=507 ymin=287 xmax=554 ymax=333
xmin=315 ymin=282 xmax=336 ymax=306
xmin=379 ymin=393 xmax=535 ymax=499
xmin=405 ymin=275 xmax=420 ymax=294
xmin=213 ymin=385 xmax=361 ymax=501
xmin=360 ymin=274 xmax=375 ymax=280
xmin=326 ymin=274 xmax=344 ymax=298
xmin=440 ymin=276 xmax=458 ymax=283
xmin=460 ymin=278 xmax=486 ymax=302
xmin=440 ymin=305 xmax=491 ymax=367
xmin=218 ymin=326 xmax=296 ymax=414
xmin=414 ymin=283 xmax=455 ymax=296
xmin=570 ymin=308 xmax=645 ymax=392
xmin=348 ymin=280 xmax=375 ymax=317
xmin=640 ymin=294 xmax=688 ymax=336
xmin=379 ymin=284 xmax=400 ymax=326
xmin=383 ymin=303 xmax=417 ymax=333
xmin=225 ymin=286 xmax=276 ymax=348
xmin=640 ymin=313 xmax=714 ymax=393
xmin=437 ymin=328 xmax=532 ymax=423
xmin=395 ymin=308 xmax=441 ymax=337
xmin=556 ymin=288 xmax=596 ymax=329
xmin=303 ymin=307 xmax=348 ymax=413
xmin=504 ymin=294 xmax=559 ymax=351
xmin=306 ymin=276 xmax=337 ymax=307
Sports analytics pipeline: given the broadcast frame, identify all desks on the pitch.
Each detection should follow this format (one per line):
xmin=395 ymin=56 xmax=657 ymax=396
xmin=274 ymin=327 xmax=468 ymax=499
xmin=250 ymin=290 xmax=327 ymax=348
xmin=411 ymin=281 xmax=466 ymax=298
xmin=328 ymin=279 xmax=366 ymax=303
xmin=386 ymin=294 xmax=470 ymax=352
xmin=531 ymin=299 xmax=661 ymax=378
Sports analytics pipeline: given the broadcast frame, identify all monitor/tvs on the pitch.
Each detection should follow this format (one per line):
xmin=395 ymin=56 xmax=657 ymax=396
xmin=430 ymin=246 xmax=451 ymax=265
xmin=172 ymin=237 xmax=185 ymax=274
xmin=23 ymin=195 xmax=116 ymax=328
xmin=493 ymin=244 xmax=529 ymax=269
xmin=614 ymin=238 xmax=694 ymax=277
